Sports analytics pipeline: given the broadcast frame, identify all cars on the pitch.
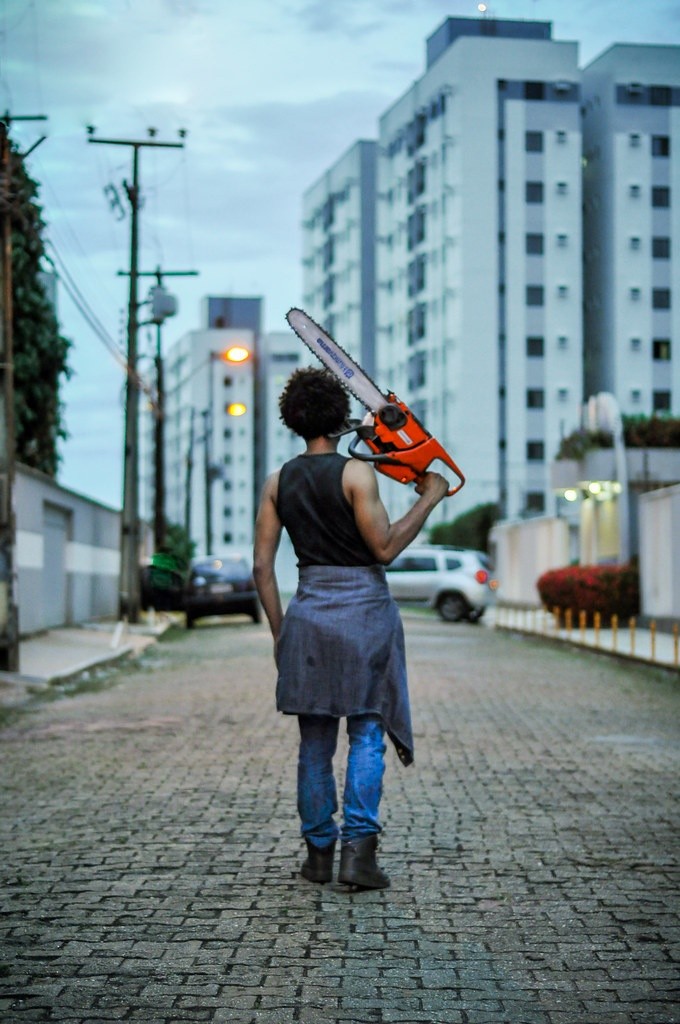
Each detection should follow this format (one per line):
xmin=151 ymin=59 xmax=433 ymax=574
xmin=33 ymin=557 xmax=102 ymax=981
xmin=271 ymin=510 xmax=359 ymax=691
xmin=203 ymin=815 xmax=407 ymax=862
xmin=184 ymin=552 xmax=262 ymax=630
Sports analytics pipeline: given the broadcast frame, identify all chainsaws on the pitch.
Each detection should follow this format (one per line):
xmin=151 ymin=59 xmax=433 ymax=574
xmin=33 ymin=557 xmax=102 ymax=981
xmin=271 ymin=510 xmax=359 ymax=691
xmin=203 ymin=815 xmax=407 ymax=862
xmin=285 ymin=304 xmax=465 ymax=497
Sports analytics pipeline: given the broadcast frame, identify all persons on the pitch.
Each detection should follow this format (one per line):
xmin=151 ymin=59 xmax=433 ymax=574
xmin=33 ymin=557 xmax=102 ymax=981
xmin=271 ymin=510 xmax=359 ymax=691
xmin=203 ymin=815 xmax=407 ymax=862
xmin=251 ymin=369 xmax=450 ymax=890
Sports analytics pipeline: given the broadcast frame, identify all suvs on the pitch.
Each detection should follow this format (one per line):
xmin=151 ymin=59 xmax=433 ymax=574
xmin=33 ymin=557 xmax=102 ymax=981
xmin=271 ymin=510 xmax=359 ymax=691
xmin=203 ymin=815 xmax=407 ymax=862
xmin=385 ymin=546 xmax=498 ymax=622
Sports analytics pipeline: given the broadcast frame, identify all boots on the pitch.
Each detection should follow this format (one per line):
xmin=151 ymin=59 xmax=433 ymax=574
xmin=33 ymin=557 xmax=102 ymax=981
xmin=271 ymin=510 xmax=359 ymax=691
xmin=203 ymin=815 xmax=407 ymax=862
xmin=337 ymin=834 xmax=390 ymax=889
xmin=300 ymin=837 xmax=336 ymax=884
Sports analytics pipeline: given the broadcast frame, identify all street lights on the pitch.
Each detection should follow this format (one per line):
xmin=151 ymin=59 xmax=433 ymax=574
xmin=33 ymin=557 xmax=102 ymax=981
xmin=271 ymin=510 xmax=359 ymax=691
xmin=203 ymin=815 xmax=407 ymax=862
xmin=151 ymin=345 xmax=253 ymax=556
